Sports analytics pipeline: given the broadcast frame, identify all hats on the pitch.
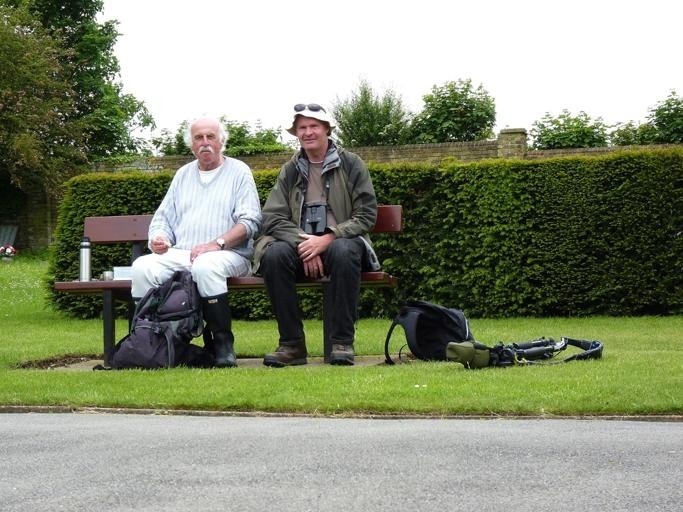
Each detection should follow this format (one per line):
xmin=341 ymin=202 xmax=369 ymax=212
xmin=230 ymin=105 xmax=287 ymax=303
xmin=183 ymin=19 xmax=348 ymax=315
xmin=286 ymin=103 xmax=336 ymax=136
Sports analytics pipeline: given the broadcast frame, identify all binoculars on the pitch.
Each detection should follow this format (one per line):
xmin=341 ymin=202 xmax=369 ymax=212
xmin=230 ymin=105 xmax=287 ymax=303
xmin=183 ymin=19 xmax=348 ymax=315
xmin=514 ymin=337 xmax=555 ymax=360
xmin=305 ymin=202 xmax=327 ymax=235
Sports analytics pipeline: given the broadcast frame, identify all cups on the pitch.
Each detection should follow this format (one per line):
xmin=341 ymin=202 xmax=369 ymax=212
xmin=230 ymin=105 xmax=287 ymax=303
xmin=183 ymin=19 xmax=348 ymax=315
xmin=103 ymin=271 xmax=114 ymax=282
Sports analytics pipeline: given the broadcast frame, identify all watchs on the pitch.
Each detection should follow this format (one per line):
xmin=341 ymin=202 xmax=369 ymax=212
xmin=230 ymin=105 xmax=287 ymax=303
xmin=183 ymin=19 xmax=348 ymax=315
xmin=215 ymin=237 xmax=225 ymax=250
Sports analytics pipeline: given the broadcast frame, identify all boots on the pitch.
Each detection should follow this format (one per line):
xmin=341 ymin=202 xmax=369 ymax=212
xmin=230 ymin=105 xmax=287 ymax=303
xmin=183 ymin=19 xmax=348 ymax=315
xmin=201 ymin=292 xmax=237 ymax=367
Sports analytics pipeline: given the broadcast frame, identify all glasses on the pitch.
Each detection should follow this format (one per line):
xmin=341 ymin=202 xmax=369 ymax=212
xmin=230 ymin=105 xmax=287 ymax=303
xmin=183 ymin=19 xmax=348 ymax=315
xmin=294 ymin=104 xmax=326 ymax=114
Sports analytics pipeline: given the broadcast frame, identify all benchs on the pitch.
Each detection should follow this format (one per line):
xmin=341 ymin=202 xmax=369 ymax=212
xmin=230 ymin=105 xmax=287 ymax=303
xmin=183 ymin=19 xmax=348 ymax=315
xmin=53 ymin=203 xmax=405 ymax=370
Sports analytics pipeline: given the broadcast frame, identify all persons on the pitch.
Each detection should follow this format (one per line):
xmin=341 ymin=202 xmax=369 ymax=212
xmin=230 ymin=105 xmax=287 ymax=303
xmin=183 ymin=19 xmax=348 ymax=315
xmin=130 ymin=116 xmax=262 ymax=367
xmin=249 ymin=103 xmax=382 ymax=369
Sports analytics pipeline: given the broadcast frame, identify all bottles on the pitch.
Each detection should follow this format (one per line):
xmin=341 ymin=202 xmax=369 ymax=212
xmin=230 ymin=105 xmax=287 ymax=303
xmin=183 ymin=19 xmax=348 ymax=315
xmin=79 ymin=235 xmax=92 ymax=282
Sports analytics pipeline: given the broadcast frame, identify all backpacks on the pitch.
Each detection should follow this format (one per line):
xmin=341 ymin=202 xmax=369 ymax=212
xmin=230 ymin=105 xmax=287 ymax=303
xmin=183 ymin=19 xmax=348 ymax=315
xmin=384 ymin=299 xmax=474 ymax=365
xmin=92 ymin=270 xmax=208 ymax=370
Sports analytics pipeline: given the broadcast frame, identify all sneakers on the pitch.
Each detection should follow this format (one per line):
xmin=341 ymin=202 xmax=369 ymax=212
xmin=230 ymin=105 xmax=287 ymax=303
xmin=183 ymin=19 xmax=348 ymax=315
xmin=330 ymin=344 xmax=354 ymax=365
xmin=263 ymin=345 xmax=307 ymax=367
